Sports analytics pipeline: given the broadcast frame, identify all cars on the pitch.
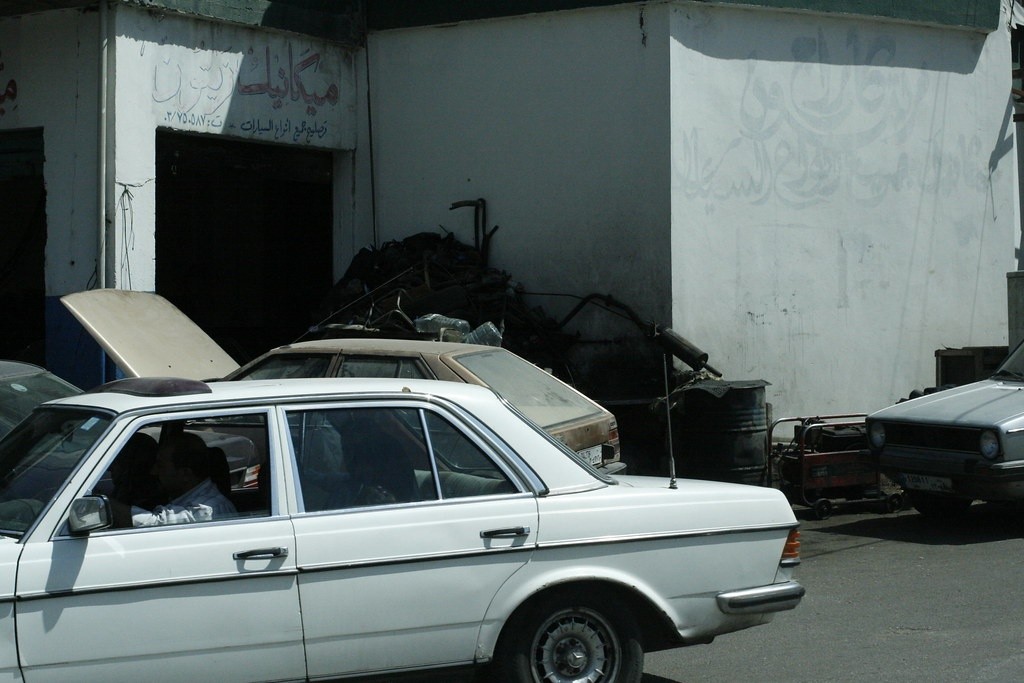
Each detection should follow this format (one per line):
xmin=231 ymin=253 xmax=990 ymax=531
xmin=0 ymin=374 xmax=804 ymax=683
xmin=56 ymin=289 xmax=628 ymax=484
xmin=0 ymin=362 xmax=261 ymax=501
xmin=864 ymin=335 xmax=1023 ymax=524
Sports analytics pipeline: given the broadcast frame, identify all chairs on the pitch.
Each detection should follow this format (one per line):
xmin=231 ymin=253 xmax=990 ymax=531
xmin=207 ymin=447 xmax=232 ymax=497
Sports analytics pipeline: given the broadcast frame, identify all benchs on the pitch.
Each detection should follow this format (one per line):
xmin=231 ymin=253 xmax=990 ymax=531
xmin=414 ymin=470 xmax=513 ymax=498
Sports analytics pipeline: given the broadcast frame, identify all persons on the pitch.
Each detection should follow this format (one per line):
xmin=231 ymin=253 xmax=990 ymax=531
xmin=108 ymin=432 xmax=238 ymax=526
